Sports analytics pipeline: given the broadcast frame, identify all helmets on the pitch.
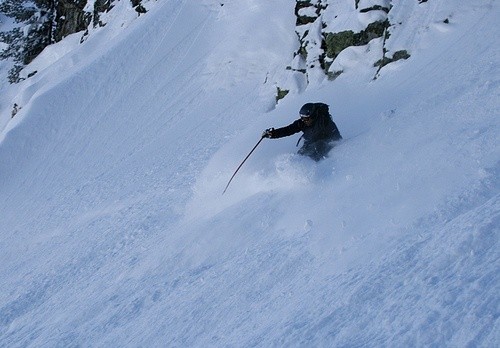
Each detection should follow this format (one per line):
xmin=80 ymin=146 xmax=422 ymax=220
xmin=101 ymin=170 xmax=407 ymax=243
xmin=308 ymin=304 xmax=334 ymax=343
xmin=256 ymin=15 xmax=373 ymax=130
xmin=299 ymin=102 xmax=316 ymax=117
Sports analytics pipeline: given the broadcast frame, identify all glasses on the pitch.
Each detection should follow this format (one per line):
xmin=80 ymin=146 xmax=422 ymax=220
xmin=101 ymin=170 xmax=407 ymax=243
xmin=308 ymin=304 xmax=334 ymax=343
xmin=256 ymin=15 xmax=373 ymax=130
xmin=300 ymin=116 xmax=311 ymax=121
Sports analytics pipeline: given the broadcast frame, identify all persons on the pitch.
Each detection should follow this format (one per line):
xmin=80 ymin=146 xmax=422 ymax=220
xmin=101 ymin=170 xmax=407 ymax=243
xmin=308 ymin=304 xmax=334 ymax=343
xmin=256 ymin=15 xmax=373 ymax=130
xmin=262 ymin=100 xmax=342 ymax=162
